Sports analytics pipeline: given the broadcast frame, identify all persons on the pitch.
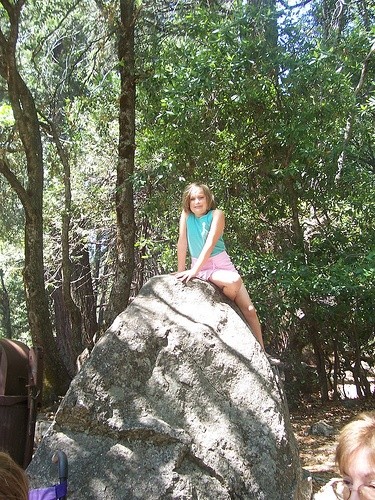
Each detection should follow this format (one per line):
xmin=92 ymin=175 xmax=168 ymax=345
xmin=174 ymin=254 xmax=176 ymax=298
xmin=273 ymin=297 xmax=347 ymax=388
xmin=0 ymin=450 xmax=29 ymax=500
xmin=177 ymin=183 xmax=282 ymax=365
xmin=312 ymin=410 xmax=374 ymax=499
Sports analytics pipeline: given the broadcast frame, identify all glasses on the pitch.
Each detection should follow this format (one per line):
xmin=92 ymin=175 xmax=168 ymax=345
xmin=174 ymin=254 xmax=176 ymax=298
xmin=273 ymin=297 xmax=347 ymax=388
xmin=332 ymin=481 xmax=375 ymax=500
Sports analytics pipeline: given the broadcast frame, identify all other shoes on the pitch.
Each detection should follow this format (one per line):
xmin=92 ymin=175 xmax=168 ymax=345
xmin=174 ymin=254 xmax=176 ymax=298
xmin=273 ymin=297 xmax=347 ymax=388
xmin=266 ymin=353 xmax=280 ymax=364
